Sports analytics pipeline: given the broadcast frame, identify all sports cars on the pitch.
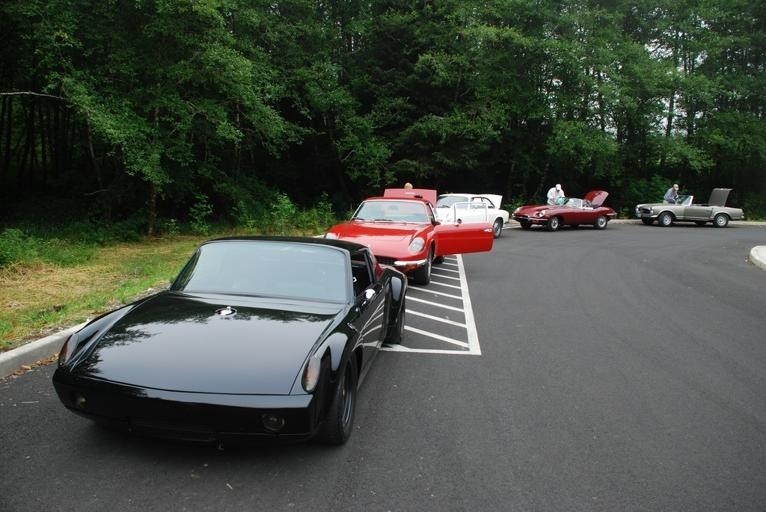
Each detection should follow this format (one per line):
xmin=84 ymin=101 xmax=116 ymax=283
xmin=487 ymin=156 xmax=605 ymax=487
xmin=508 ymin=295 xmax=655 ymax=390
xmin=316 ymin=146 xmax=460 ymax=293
xmin=512 ymin=190 xmax=617 ymax=231
xmin=51 ymin=235 xmax=407 ymax=446
xmin=325 ymin=188 xmax=495 ymax=287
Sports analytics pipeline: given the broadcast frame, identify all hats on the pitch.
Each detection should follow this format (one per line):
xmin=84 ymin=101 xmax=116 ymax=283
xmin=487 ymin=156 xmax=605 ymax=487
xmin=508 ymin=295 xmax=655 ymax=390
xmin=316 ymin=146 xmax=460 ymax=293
xmin=673 ymin=183 xmax=680 ymax=192
xmin=556 ymin=184 xmax=562 ymax=192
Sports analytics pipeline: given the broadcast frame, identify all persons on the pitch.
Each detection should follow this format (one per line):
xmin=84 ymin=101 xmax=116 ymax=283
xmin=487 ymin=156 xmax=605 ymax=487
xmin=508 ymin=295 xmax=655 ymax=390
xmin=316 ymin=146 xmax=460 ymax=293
xmin=664 ymin=184 xmax=679 ymax=204
xmin=547 ymin=184 xmax=565 ymax=206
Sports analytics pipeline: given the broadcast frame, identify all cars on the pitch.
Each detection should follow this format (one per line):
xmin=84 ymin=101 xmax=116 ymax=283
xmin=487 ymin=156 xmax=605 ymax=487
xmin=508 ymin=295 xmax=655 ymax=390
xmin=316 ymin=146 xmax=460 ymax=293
xmin=435 ymin=194 xmax=509 ymax=239
xmin=635 ymin=188 xmax=745 ymax=228
